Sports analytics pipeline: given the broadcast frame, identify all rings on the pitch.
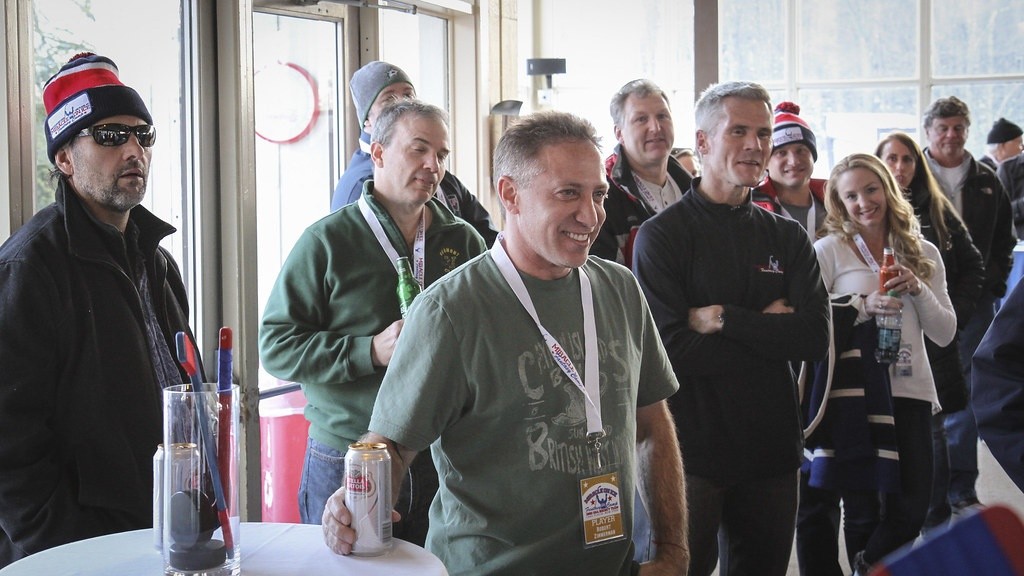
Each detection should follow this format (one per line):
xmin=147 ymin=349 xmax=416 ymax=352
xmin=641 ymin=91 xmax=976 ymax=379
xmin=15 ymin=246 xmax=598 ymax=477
xmin=879 ymin=301 xmax=882 ymax=309
xmin=905 ymin=280 xmax=910 ymax=289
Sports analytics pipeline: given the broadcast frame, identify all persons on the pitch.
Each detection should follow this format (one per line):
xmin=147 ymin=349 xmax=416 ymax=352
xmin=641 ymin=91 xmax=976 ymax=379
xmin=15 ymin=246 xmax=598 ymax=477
xmin=333 ymin=62 xmax=501 ymax=249
xmin=0 ymin=52 xmax=208 ymax=569
xmin=259 ymin=102 xmax=486 ymax=548
xmin=322 ymin=113 xmax=690 ymax=576
xmin=632 ymin=81 xmax=829 ymax=576
xmin=753 ymin=95 xmax=1024 ymax=576
xmin=589 ymin=80 xmax=699 ymax=269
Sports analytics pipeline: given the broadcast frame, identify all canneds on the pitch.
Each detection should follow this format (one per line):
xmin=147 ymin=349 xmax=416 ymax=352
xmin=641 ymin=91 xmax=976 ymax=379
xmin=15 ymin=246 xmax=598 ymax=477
xmin=343 ymin=442 xmax=392 ymax=557
xmin=153 ymin=442 xmax=200 ymax=551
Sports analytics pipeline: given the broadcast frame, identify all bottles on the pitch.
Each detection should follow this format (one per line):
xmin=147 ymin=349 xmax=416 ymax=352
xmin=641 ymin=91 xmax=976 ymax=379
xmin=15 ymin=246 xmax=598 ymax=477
xmin=881 ymin=247 xmax=899 ymax=295
xmin=876 ymin=291 xmax=903 ymax=365
xmin=395 ymin=256 xmax=423 ymax=320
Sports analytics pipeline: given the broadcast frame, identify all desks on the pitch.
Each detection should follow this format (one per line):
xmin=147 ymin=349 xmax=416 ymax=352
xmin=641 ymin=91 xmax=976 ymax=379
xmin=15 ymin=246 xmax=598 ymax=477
xmin=0 ymin=521 xmax=450 ymax=576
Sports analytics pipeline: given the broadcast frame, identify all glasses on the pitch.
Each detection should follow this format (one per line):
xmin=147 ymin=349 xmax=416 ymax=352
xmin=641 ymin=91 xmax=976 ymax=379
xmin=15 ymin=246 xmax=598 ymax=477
xmin=76 ymin=123 xmax=156 ymax=147
xmin=618 ymin=80 xmax=656 ymax=94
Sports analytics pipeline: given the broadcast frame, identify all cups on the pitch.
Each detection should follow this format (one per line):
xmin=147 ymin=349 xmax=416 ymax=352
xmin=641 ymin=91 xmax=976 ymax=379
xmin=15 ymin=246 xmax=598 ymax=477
xmin=164 ymin=381 xmax=242 ymax=575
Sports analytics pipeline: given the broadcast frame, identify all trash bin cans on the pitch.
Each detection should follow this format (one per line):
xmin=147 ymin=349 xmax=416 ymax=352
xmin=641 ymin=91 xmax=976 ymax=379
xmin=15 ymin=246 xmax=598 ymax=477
xmin=231 ymin=387 xmax=307 ymax=522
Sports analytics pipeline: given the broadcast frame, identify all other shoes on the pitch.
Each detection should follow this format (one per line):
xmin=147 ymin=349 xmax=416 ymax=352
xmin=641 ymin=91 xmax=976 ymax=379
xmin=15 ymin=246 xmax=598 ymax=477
xmin=854 ymin=551 xmax=869 ymax=575
xmin=951 ymin=500 xmax=985 ymax=516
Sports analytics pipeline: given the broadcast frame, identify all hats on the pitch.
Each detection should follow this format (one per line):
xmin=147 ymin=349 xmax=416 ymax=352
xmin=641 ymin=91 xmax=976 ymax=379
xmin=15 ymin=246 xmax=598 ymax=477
xmin=350 ymin=61 xmax=415 ymax=131
xmin=44 ymin=52 xmax=153 ymax=164
xmin=987 ymin=118 xmax=1023 ymax=144
xmin=772 ymin=102 xmax=818 ymax=162
